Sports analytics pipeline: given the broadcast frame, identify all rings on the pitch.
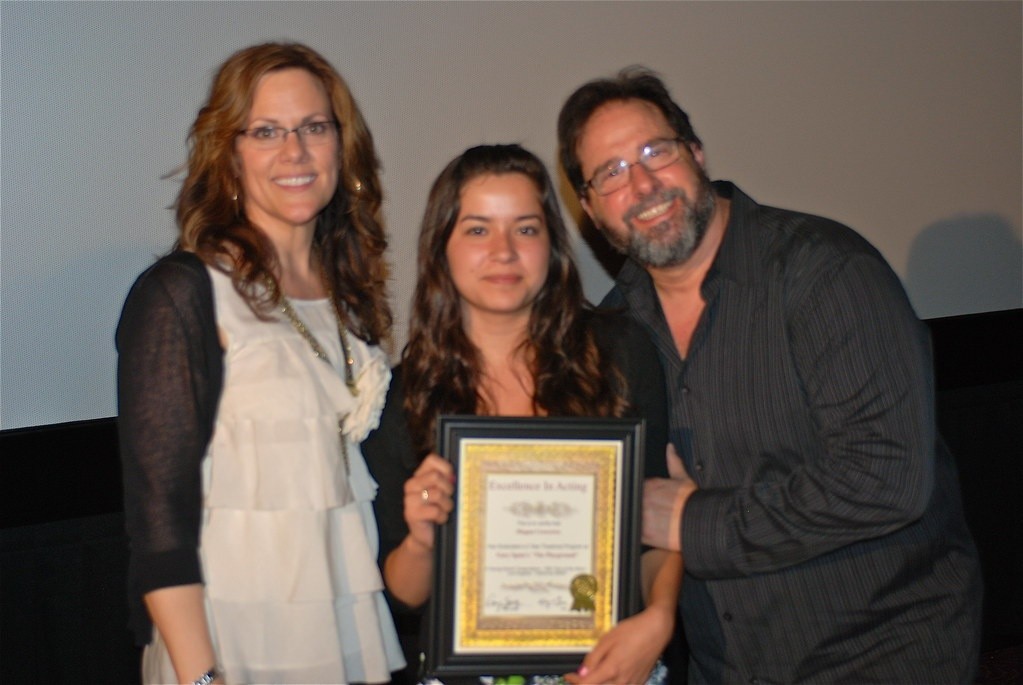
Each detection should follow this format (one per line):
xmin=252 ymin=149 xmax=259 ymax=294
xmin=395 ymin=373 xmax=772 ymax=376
xmin=423 ymin=489 xmax=429 ymax=503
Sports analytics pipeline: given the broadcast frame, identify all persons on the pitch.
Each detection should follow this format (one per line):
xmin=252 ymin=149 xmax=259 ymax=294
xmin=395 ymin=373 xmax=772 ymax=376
xmin=116 ymin=41 xmax=408 ymax=685
xmin=359 ymin=143 xmax=682 ymax=685
xmin=556 ymin=62 xmax=987 ymax=685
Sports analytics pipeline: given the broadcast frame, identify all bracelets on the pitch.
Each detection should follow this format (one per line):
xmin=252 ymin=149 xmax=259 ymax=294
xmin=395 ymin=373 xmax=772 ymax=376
xmin=193 ymin=667 xmax=218 ymax=685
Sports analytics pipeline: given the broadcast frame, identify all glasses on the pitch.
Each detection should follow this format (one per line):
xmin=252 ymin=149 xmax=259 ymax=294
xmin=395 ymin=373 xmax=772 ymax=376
xmin=582 ymin=135 xmax=685 ymax=196
xmin=238 ymin=120 xmax=339 ymax=148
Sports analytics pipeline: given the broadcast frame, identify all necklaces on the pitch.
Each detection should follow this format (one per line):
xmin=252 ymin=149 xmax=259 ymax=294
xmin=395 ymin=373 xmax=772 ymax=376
xmin=262 ymin=267 xmax=357 ymax=476
